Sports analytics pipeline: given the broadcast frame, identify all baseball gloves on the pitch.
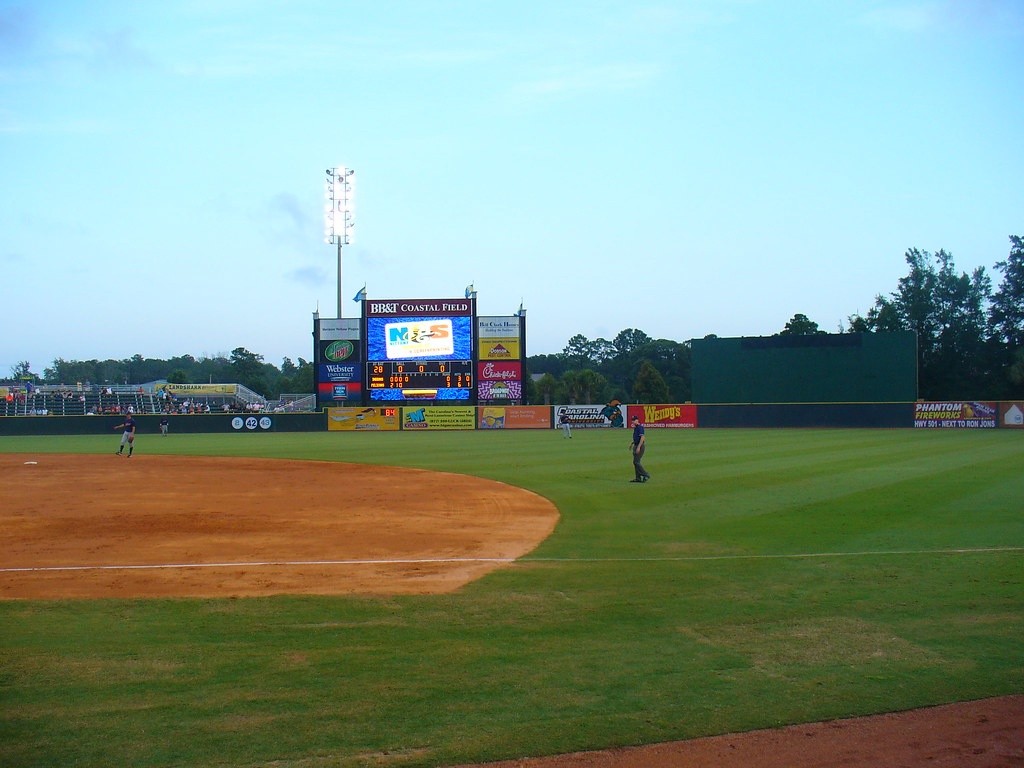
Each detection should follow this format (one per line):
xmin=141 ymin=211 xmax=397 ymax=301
xmin=128 ymin=435 xmax=134 ymax=443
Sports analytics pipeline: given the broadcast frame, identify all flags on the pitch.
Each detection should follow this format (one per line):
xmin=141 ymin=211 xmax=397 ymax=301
xmin=353 ymin=287 xmax=367 ymax=302
xmin=465 ymin=284 xmax=473 ymax=298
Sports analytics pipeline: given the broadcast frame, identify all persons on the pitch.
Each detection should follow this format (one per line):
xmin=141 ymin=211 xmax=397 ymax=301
xmin=628 ymin=415 xmax=651 ymax=483
xmin=560 ymin=411 xmax=571 ymax=439
xmin=4 ymin=381 xmax=293 ymax=415
xmin=160 ymin=416 xmax=168 ymax=437
xmin=113 ymin=412 xmax=136 ymax=458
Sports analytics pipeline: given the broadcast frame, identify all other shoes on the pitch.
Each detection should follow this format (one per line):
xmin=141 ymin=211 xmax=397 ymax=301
xmin=116 ymin=452 xmax=122 ymax=456
xmin=629 ymin=479 xmax=641 ymax=482
xmin=128 ymin=455 xmax=132 ymax=457
xmin=643 ymin=476 xmax=649 ymax=482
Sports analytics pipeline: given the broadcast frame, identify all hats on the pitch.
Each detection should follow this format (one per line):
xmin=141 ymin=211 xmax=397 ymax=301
xmin=126 ymin=410 xmax=131 ymax=415
xmin=632 ymin=416 xmax=637 ymax=421
